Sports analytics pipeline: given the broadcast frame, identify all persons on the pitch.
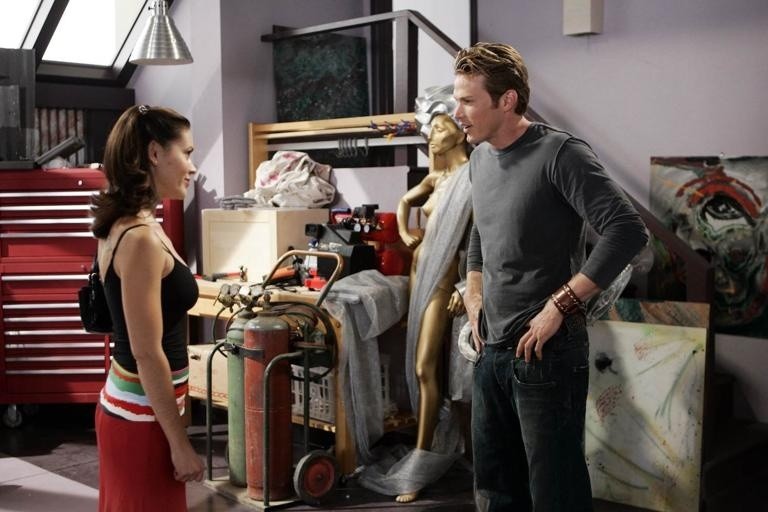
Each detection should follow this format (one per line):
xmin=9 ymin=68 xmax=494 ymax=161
xmin=452 ymin=41 xmax=652 ymax=512
xmin=395 ymin=111 xmax=472 ymax=502
xmin=94 ymin=103 xmax=207 ymax=512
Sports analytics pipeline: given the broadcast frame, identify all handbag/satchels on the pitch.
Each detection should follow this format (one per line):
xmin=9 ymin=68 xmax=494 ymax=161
xmin=78 ymin=262 xmax=114 ymax=333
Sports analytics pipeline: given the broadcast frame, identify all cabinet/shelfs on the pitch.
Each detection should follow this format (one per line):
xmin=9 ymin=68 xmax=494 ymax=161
xmin=189 ymin=282 xmax=415 ymax=476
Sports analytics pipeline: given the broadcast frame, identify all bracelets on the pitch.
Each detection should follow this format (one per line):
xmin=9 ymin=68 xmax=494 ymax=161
xmin=551 ymin=283 xmax=581 ymax=316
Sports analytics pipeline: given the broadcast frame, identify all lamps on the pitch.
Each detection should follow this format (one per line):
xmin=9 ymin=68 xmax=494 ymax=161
xmin=127 ymin=0 xmax=196 ymax=68
